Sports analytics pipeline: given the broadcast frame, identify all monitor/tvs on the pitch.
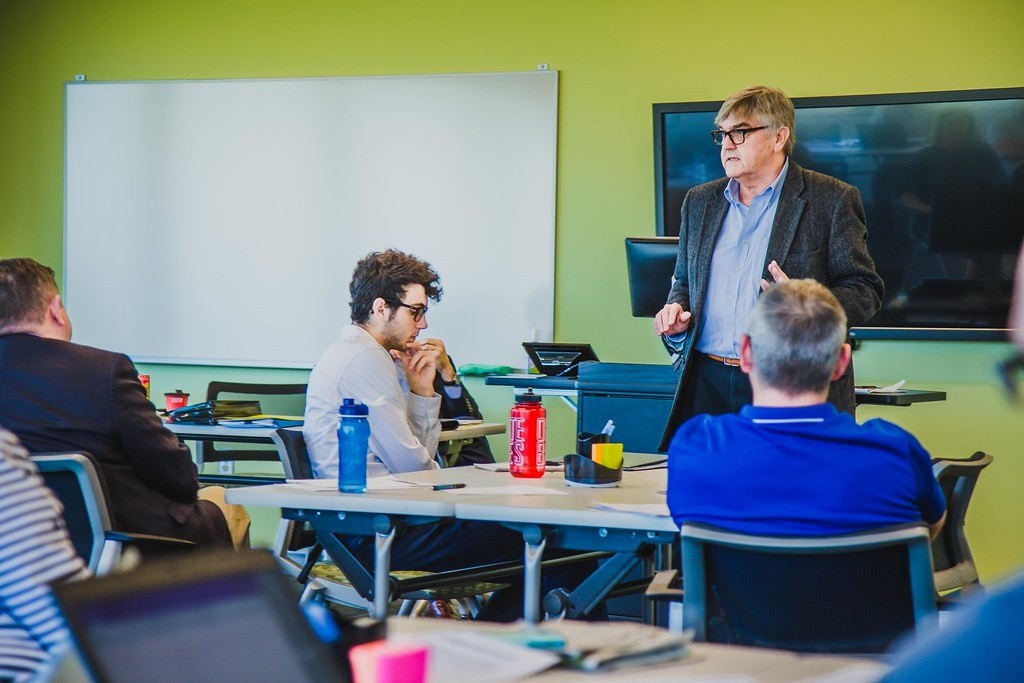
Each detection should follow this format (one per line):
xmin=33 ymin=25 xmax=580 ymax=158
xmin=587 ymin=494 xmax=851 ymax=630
xmin=652 ymin=87 xmax=1024 ymax=341
xmin=523 ymin=342 xmax=602 ymax=378
xmin=625 ymin=236 xmax=683 ymax=318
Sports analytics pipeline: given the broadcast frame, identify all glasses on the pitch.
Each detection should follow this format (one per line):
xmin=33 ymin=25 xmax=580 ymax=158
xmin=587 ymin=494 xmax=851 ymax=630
xmin=994 ymin=353 xmax=1024 ymax=393
xmin=370 ymin=298 xmax=427 ymax=322
xmin=710 ymin=124 xmax=788 ymax=145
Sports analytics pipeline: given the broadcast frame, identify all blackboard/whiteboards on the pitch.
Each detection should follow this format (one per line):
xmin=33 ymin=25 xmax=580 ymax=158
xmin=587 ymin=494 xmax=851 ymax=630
xmin=61 ymin=71 xmax=561 ymax=371
xmin=655 ymin=85 xmax=1024 ymax=341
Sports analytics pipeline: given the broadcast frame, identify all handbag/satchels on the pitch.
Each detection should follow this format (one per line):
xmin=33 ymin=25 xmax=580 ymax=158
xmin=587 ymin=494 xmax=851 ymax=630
xmin=160 ymin=399 xmax=262 ymax=425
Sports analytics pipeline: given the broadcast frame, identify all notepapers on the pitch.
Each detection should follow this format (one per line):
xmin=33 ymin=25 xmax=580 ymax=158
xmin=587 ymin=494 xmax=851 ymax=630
xmin=591 ymin=443 xmax=623 ymax=470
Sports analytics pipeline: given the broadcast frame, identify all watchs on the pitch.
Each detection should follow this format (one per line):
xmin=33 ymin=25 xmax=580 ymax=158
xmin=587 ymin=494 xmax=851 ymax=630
xmin=443 ymin=372 xmax=461 ymax=386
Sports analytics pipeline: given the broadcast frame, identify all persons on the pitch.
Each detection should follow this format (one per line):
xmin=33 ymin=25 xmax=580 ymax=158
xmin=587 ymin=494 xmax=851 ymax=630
xmin=865 ymin=241 xmax=1024 ymax=683
xmin=0 ymin=426 xmax=92 ymax=683
xmin=0 ymin=258 xmax=251 ymax=570
xmin=665 ymin=278 xmax=947 ymax=543
xmin=652 ymin=84 xmax=884 ymax=456
xmin=302 ymin=249 xmax=609 ymax=624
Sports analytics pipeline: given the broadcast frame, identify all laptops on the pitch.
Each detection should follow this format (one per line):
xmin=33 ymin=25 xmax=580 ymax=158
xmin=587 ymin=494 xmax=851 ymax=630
xmin=46 ymin=545 xmax=355 ymax=683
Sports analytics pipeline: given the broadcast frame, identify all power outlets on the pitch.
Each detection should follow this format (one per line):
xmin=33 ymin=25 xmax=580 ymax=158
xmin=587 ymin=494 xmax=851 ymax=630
xmin=219 ymin=447 xmax=234 ymax=474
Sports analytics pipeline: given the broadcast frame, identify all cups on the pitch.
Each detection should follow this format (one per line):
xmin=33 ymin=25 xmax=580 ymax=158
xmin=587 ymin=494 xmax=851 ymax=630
xmin=162 ymin=390 xmax=190 ymax=423
xmin=591 ymin=442 xmax=623 ymax=469
xmin=576 ymin=431 xmax=611 ymax=458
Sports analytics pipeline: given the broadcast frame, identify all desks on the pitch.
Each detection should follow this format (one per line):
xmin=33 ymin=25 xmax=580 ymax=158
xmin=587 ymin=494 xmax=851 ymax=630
xmin=461 ymin=467 xmax=679 ymax=624
xmin=353 ymin=617 xmax=893 ymax=683
xmin=161 ymin=415 xmax=303 ymax=439
xmin=226 ymin=467 xmax=634 ymax=620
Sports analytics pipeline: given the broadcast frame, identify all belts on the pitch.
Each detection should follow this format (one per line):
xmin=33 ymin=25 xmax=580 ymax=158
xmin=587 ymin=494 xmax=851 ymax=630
xmin=707 ymin=354 xmax=741 ymax=365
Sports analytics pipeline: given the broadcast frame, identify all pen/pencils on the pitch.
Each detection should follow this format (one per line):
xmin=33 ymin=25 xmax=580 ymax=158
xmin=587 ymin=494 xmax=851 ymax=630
xmin=528 ymin=640 xmax=564 ymax=648
xmin=601 ymin=420 xmax=616 ymax=436
xmin=433 ymin=483 xmax=466 ymax=490
xmin=546 ymin=460 xmax=558 ymax=466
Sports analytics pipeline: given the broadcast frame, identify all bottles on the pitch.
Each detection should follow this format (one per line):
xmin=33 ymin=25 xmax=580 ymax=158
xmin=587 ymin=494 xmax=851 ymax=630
xmin=337 ymin=396 xmax=386 ymax=494
xmin=510 ymin=387 xmax=546 ymax=478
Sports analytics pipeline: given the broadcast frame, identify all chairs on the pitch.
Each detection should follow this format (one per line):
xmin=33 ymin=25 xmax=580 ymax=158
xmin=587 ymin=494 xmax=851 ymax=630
xmin=275 ymin=428 xmax=512 ymax=618
xmin=931 ymin=450 xmax=994 ymax=597
xmin=192 ymin=381 xmax=308 ymax=474
xmin=269 ymin=427 xmax=314 ymax=480
xmin=33 ymin=449 xmax=199 ymax=577
xmin=676 ymin=520 xmax=940 ymax=655
